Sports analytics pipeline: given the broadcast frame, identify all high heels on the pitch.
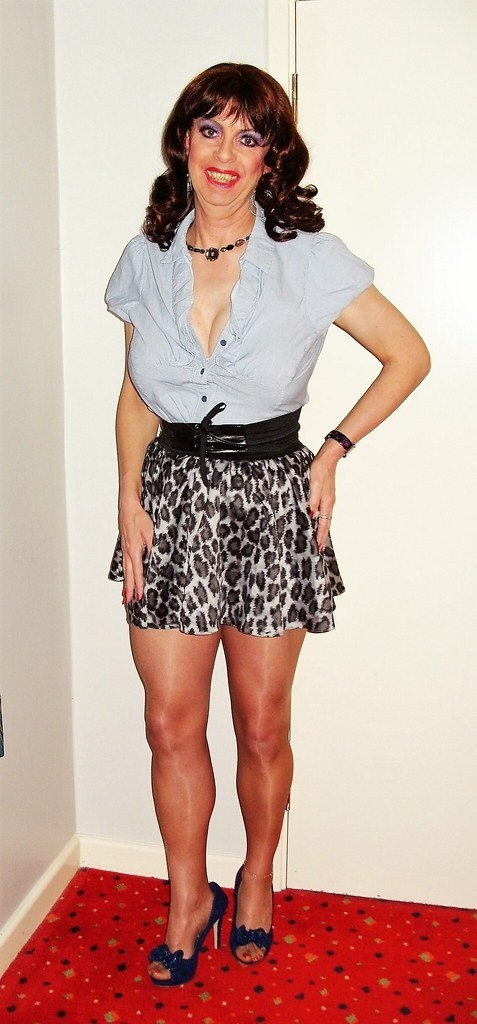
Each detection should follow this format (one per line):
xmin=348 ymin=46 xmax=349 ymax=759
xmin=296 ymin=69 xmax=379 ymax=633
xmin=147 ymin=880 xmax=228 ymax=987
xmin=230 ymin=864 xmax=274 ymax=964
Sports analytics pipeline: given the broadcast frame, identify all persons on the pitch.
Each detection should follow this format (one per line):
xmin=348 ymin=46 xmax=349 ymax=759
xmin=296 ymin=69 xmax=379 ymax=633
xmin=104 ymin=63 xmax=431 ymax=986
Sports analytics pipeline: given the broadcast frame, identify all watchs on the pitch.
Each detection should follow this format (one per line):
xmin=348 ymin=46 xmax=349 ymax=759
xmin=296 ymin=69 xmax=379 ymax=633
xmin=324 ymin=430 xmax=355 ymax=458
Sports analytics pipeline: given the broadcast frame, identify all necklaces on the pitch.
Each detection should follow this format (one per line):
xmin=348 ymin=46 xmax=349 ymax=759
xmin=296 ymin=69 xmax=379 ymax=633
xmin=185 ymin=218 xmax=251 ymax=261
